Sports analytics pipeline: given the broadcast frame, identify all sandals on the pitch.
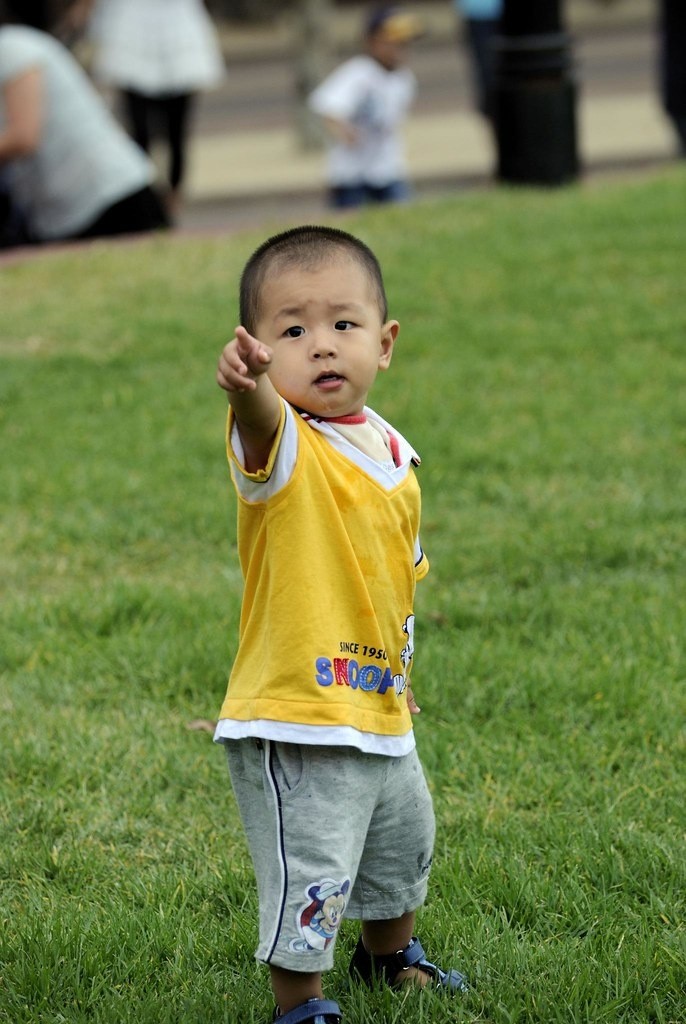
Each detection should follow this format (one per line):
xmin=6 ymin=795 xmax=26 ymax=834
xmin=272 ymin=997 xmax=343 ymax=1024
xmin=349 ymin=934 xmax=484 ymax=1023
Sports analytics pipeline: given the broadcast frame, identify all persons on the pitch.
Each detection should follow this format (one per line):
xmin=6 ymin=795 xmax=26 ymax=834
xmin=0 ymin=24 xmax=171 ymax=245
xmin=213 ymin=225 xmax=464 ymax=1024
xmin=311 ymin=10 xmax=424 ymax=209
xmin=92 ymin=0 xmax=227 ymax=187
